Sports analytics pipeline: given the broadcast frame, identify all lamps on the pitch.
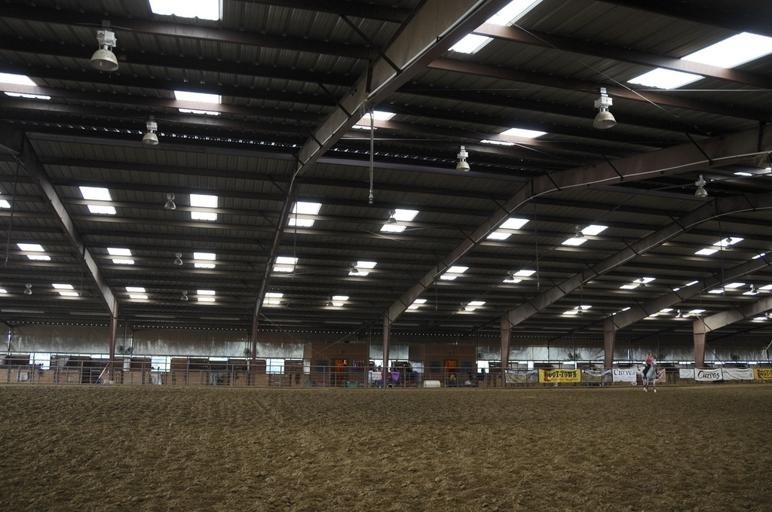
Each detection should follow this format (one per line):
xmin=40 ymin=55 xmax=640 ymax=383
xmin=142 ymin=119 xmax=160 ymax=146
xmin=593 ymin=87 xmax=618 ymax=129
xmin=163 ymin=193 xmax=177 ymax=210
xmin=24 ymin=283 xmax=33 ymax=296
xmin=90 ymin=28 xmax=119 ymax=72
xmin=181 ymin=290 xmax=188 ymax=301
xmin=455 ymin=145 xmax=470 ymax=171
xmin=694 ymin=174 xmax=708 ymax=199
xmin=174 ymin=252 xmax=184 ymax=266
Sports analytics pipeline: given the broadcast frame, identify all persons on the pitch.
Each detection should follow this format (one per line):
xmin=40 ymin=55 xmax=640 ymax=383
xmin=642 ymin=352 xmax=658 ymax=378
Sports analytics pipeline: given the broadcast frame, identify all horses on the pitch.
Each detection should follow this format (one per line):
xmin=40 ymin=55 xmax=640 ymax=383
xmin=641 ymin=359 xmax=657 ymax=392
